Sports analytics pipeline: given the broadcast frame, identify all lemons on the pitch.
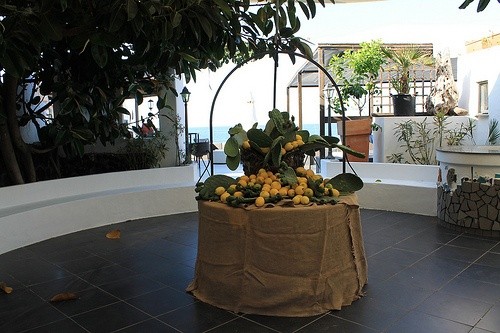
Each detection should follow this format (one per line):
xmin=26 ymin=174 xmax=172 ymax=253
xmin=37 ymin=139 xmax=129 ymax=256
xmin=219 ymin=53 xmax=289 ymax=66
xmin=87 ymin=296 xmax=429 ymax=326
xmin=215 ymin=167 xmax=339 ymax=207
xmin=243 ymin=135 xmax=305 ymax=155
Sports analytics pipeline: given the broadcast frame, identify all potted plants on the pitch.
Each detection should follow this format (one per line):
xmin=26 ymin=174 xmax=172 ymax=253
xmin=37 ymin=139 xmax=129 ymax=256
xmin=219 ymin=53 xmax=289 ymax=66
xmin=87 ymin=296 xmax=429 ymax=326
xmin=381 ymin=43 xmax=438 ymax=116
xmin=322 ymin=39 xmax=391 ymax=163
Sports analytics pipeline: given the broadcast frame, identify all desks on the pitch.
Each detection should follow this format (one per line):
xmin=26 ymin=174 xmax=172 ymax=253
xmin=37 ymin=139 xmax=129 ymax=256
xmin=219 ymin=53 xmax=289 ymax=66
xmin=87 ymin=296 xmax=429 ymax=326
xmin=187 ymin=193 xmax=369 ymax=318
xmin=189 ymin=142 xmax=219 ymax=163
